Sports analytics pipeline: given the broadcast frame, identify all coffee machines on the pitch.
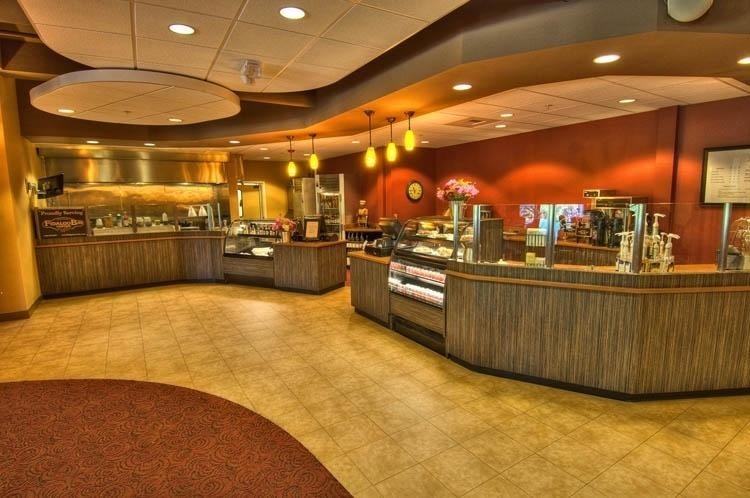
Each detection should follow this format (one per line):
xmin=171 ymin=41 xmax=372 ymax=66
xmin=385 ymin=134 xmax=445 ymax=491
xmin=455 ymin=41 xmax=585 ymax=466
xmin=594 ymin=195 xmax=647 ymax=247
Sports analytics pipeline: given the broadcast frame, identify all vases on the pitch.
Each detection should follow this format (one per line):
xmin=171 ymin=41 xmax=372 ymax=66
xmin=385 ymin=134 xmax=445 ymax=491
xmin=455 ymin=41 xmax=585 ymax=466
xmin=282 ymin=230 xmax=290 ymax=243
xmin=452 ymin=200 xmax=462 ymax=241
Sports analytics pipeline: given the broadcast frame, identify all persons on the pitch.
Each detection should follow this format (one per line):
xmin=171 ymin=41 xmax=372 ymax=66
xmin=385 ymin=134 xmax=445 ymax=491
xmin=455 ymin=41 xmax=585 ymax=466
xmin=356 ymin=200 xmax=368 ymax=229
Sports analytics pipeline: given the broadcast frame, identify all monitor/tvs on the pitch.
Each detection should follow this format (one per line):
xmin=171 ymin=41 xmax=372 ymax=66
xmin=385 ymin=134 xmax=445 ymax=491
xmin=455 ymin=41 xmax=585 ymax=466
xmin=378 ymin=217 xmax=402 ymax=239
xmin=38 ymin=174 xmax=64 ymax=198
xmin=303 ymin=215 xmax=326 ymax=233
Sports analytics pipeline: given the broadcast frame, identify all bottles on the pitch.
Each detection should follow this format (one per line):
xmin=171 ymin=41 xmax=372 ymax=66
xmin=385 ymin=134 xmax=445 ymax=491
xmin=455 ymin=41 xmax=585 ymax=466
xmin=347 ymin=232 xmax=368 ymax=269
xmin=238 ymin=224 xmax=279 ymax=235
xmin=96 ymin=210 xmax=169 ymax=228
xmin=188 ymin=206 xmax=208 ymax=225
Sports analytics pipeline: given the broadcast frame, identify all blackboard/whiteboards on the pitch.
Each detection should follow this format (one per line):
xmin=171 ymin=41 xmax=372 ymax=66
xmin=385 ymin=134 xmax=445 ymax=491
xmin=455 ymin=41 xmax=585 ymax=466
xmin=33 ymin=205 xmax=92 ymax=240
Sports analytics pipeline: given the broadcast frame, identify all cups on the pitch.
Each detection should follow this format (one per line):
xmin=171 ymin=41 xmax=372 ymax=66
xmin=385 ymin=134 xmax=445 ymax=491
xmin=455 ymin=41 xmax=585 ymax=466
xmin=466 ymin=248 xmax=472 ymax=260
xmin=222 ymin=220 xmax=226 ymax=227
xmin=292 ymin=231 xmax=303 ymax=241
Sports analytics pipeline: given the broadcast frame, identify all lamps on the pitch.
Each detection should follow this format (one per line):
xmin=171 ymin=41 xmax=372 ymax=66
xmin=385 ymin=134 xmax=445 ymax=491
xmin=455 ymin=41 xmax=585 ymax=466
xmin=404 ymin=109 xmax=415 ymax=153
xmin=286 ymin=134 xmax=297 ymax=178
xmin=385 ymin=117 xmax=398 ymax=165
xmin=364 ymin=109 xmax=378 ymax=170
xmin=307 ymin=132 xmax=319 ymax=172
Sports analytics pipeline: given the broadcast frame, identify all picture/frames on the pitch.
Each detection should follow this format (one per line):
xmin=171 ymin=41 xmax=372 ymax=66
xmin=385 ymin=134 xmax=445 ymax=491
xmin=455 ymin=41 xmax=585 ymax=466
xmin=696 ymin=144 xmax=750 ymax=210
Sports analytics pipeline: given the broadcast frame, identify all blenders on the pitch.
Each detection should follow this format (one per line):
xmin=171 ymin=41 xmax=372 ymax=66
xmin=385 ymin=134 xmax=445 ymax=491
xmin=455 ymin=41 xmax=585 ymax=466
xmin=575 ymin=188 xmax=617 ymax=243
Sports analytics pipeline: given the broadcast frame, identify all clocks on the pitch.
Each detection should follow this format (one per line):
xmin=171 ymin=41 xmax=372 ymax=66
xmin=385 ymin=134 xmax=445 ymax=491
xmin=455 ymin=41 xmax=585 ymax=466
xmin=405 ymin=178 xmax=424 ymax=203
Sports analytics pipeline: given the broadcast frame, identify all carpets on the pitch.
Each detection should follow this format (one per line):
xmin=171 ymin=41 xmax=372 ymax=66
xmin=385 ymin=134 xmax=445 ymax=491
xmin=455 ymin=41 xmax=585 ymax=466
xmin=1 ymin=378 xmax=356 ymax=497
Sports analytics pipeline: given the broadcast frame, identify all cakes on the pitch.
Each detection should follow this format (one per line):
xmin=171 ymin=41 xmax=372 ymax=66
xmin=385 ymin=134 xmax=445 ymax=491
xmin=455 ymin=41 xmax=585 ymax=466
xmin=414 ymin=222 xmax=474 ymax=258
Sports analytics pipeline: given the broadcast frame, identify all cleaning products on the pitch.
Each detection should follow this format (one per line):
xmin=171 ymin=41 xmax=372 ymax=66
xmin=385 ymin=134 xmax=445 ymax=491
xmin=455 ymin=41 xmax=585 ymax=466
xmin=614 ymin=211 xmax=680 ymax=272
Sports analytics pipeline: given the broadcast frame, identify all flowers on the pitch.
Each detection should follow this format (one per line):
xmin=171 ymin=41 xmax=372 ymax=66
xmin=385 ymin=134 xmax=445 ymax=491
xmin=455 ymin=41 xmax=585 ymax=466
xmin=434 ymin=176 xmax=481 ymax=203
xmin=271 ymin=212 xmax=298 ymax=232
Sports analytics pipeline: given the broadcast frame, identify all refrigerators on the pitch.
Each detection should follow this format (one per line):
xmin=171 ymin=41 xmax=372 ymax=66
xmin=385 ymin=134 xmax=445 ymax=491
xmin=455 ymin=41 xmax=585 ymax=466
xmin=315 ymin=174 xmax=362 ymax=240
